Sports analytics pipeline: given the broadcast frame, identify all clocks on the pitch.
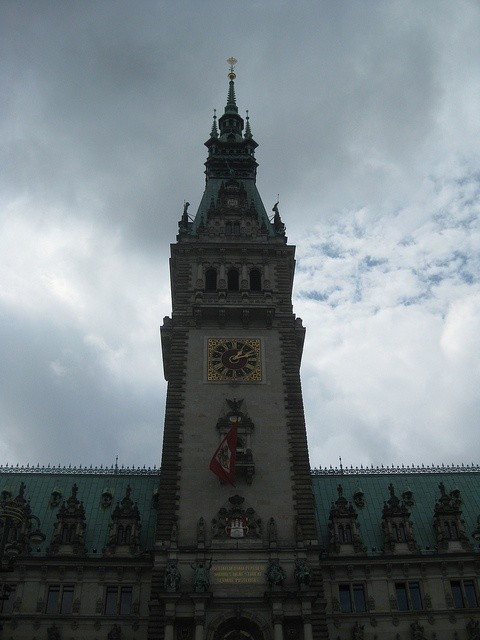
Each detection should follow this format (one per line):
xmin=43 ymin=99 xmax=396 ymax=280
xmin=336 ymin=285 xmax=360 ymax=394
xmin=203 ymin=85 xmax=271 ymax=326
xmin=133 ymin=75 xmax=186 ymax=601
xmin=207 ymin=337 xmax=262 ymax=383
